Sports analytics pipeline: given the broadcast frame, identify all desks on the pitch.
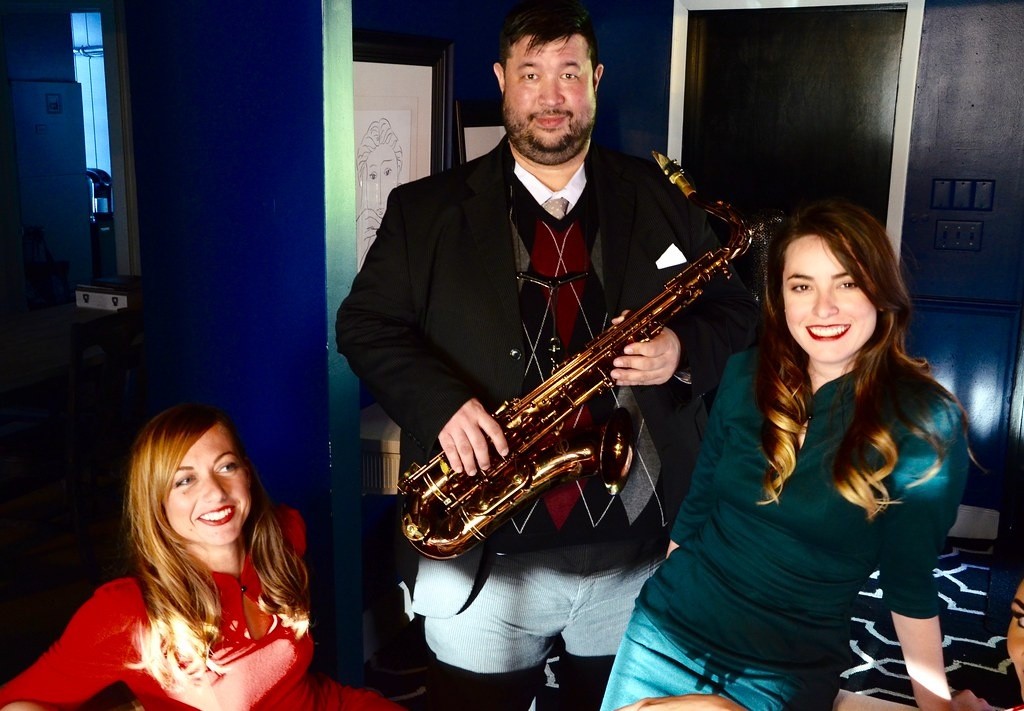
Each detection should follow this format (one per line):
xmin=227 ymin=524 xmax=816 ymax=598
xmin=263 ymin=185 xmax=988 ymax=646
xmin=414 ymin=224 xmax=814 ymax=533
xmin=0 ymin=302 xmax=119 ymax=393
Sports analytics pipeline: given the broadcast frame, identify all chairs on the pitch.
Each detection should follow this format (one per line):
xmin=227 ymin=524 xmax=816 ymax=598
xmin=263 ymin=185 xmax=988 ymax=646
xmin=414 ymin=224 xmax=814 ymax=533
xmin=24 ymin=311 xmax=147 ymax=529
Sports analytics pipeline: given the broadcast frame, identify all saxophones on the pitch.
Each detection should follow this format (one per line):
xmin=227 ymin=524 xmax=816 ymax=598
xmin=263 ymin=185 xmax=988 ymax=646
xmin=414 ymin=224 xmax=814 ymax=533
xmin=395 ymin=149 xmax=756 ymax=562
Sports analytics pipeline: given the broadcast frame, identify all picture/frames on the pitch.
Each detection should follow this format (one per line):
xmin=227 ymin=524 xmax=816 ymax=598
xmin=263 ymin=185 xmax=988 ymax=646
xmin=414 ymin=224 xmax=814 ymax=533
xmin=352 ymin=31 xmax=452 ymax=273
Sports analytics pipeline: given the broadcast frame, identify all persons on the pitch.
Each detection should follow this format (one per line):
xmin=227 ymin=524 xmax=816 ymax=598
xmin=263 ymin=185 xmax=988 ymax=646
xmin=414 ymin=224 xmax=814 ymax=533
xmin=599 ymin=201 xmax=1024 ymax=711
xmin=336 ymin=7 xmax=759 ymax=711
xmin=0 ymin=403 xmax=410 ymax=711
